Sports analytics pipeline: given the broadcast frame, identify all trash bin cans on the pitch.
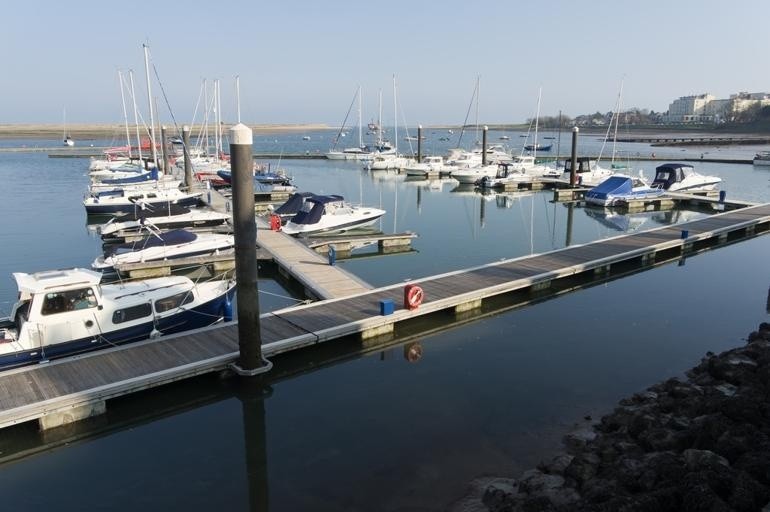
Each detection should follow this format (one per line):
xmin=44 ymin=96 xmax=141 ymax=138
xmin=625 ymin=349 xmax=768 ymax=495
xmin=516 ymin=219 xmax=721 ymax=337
xmin=720 ymin=190 xmax=726 ymax=202
xmin=272 ymin=215 xmax=281 ymax=231
xmin=327 ymin=245 xmax=336 ymax=267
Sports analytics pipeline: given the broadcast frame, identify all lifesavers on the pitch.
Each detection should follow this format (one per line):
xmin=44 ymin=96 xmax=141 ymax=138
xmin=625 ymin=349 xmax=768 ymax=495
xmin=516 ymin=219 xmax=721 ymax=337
xmin=408 ymin=344 xmax=421 ymax=362
xmin=408 ymin=287 xmax=423 ymax=307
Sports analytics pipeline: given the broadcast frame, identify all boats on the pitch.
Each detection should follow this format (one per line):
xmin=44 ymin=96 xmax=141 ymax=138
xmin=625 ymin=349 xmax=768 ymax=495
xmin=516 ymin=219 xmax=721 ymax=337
xmin=0 ymin=266 xmax=238 ymax=371
xmin=584 ymin=164 xmax=722 ymax=206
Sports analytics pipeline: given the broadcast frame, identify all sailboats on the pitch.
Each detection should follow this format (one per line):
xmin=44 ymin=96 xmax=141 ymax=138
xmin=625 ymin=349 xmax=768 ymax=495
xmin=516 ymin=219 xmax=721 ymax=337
xmin=62 ymin=100 xmax=75 ymax=146
